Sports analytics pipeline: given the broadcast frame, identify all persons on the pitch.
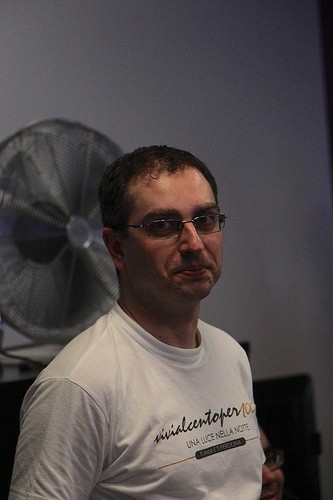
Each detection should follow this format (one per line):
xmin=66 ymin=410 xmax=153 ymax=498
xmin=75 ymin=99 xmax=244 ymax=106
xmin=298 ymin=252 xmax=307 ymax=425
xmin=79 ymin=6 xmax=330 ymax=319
xmin=255 ymin=409 xmax=292 ymax=500
xmin=8 ymin=145 xmax=267 ymax=500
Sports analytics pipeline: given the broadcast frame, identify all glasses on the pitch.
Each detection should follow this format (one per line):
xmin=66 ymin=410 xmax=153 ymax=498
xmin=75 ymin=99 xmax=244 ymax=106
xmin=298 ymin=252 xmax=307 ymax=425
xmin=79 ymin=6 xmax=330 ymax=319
xmin=115 ymin=213 xmax=228 ymax=238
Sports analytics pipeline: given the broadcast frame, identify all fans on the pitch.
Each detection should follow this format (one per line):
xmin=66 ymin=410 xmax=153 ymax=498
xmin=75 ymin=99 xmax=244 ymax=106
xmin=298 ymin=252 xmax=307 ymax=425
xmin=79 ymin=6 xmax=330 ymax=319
xmin=2 ymin=117 xmax=125 ymax=343
